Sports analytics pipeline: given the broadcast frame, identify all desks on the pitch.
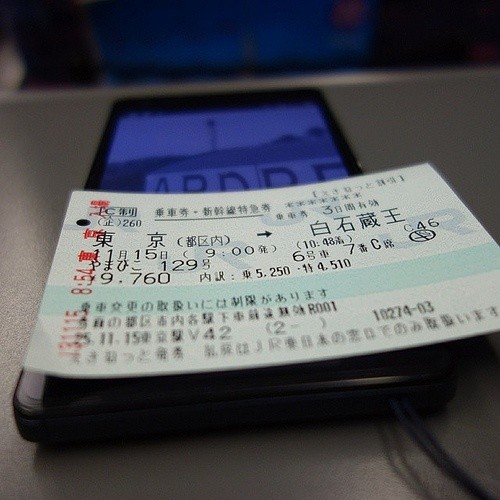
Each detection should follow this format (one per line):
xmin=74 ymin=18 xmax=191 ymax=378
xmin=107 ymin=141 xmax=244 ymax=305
xmin=3 ymin=66 xmax=500 ymax=499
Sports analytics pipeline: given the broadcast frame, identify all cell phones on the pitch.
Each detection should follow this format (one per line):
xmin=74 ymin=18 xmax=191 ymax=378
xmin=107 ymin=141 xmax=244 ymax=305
xmin=13 ymin=84 xmax=458 ymax=455
